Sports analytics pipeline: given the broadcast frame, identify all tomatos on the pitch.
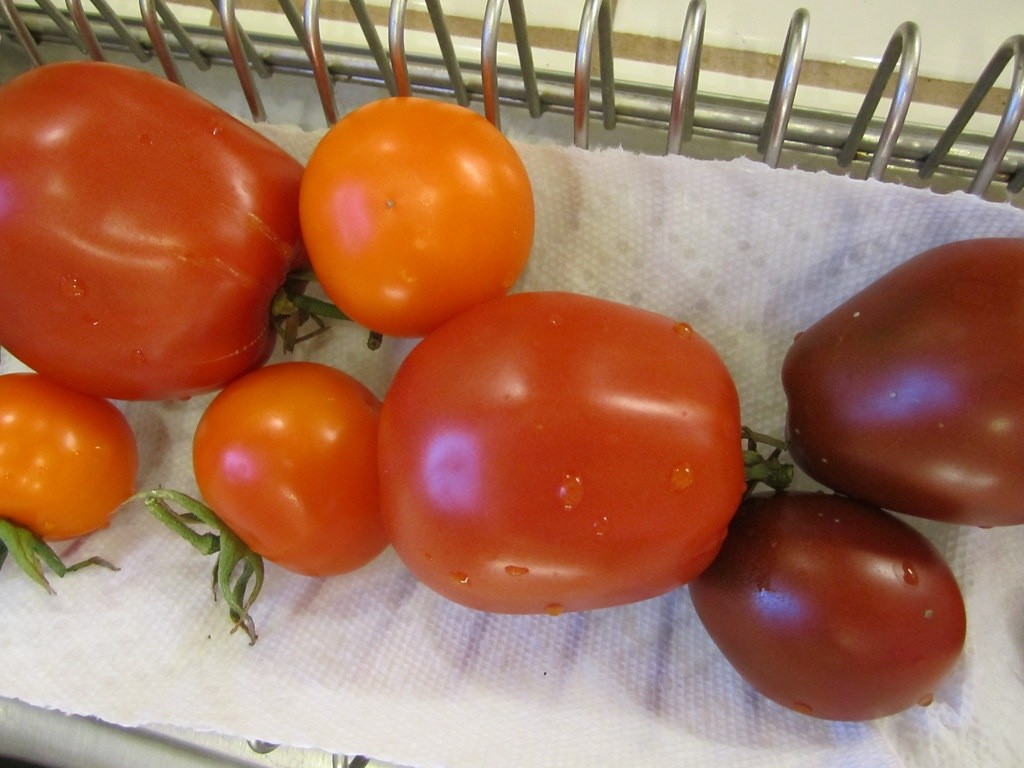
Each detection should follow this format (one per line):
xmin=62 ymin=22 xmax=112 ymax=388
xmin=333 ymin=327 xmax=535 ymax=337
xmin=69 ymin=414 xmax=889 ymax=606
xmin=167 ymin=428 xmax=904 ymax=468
xmin=0 ymin=58 xmax=1024 ymax=727
